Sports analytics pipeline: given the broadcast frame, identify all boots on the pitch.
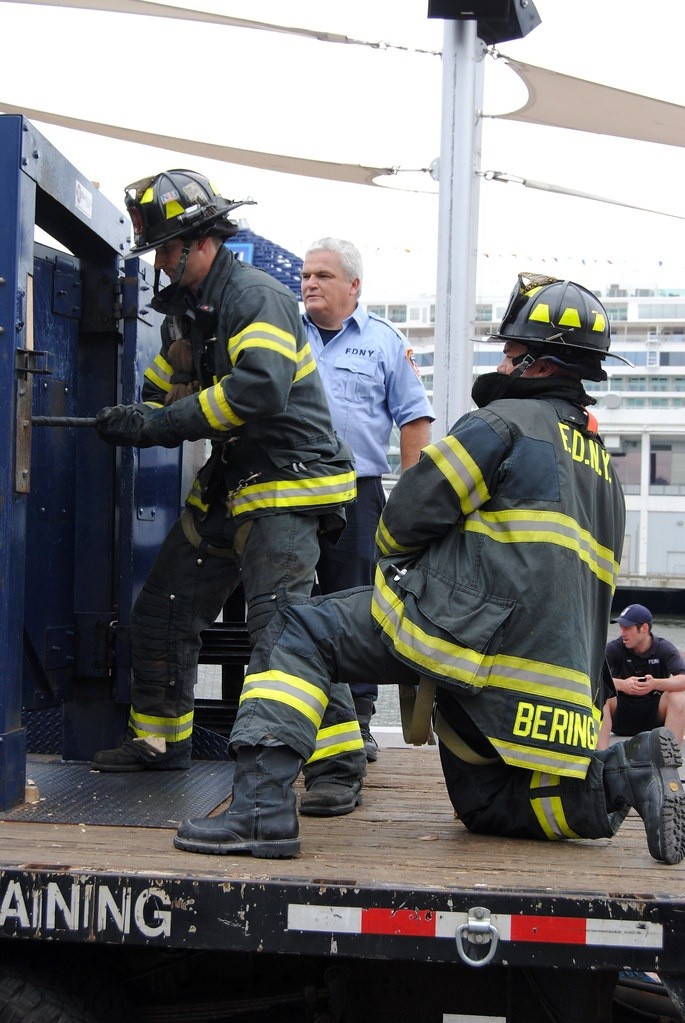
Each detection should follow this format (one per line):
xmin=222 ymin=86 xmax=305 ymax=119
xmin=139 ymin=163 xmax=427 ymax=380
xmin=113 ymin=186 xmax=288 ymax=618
xmin=593 ymin=726 xmax=685 ymax=864
xmin=173 ymin=742 xmax=305 ymax=857
xmin=353 ymin=695 xmax=379 ymax=761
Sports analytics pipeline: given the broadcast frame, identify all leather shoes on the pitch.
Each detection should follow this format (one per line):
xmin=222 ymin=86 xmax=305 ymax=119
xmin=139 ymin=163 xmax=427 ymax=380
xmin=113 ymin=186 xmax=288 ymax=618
xmin=299 ymin=776 xmax=365 ymax=815
xmin=91 ymin=730 xmax=191 ymax=772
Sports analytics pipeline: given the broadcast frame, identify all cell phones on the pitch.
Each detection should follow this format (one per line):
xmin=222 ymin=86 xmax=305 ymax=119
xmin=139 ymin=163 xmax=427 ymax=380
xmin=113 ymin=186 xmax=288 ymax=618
xmin=638 ymin=677 xmax=646 ymax=682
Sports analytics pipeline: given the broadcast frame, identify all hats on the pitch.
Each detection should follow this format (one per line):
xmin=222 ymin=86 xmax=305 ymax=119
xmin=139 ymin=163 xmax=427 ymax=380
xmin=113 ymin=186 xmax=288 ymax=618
xmin=610 ymin=603 xmax=652 ymax=629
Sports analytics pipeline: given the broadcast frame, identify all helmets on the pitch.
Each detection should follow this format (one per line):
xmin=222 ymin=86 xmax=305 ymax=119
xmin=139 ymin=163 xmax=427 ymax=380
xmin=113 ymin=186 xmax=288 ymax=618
xmin=470 ymin=273 xmax=636 ymax=369
xmin=119 ymin=168 xmax=258 ymax=263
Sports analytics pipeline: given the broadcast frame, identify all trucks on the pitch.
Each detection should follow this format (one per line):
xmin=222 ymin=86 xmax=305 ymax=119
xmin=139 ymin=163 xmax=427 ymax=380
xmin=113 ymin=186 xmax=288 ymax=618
xmin=0 ymin=113 xmax=684 ymax=1023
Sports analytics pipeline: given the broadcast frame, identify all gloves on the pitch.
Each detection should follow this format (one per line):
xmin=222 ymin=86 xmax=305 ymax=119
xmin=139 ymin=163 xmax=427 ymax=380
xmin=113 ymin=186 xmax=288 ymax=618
xmin=101 ymin=408 xmax=180 ymax=449
xmin=93 ymin=404 xmax=147 ymax=435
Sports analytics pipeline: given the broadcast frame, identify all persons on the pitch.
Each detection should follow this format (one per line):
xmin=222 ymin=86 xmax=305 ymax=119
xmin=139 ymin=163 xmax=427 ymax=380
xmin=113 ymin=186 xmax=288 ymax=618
xmin=297 ymin=237 xmax=435 ymax=762
xmin=175 ymin=272 xmax=685 ymax=865
xmin=597 ymin=604 xmax=685 ymax=751
xmin=96 ymin=168 xmax=369 ymax=816
